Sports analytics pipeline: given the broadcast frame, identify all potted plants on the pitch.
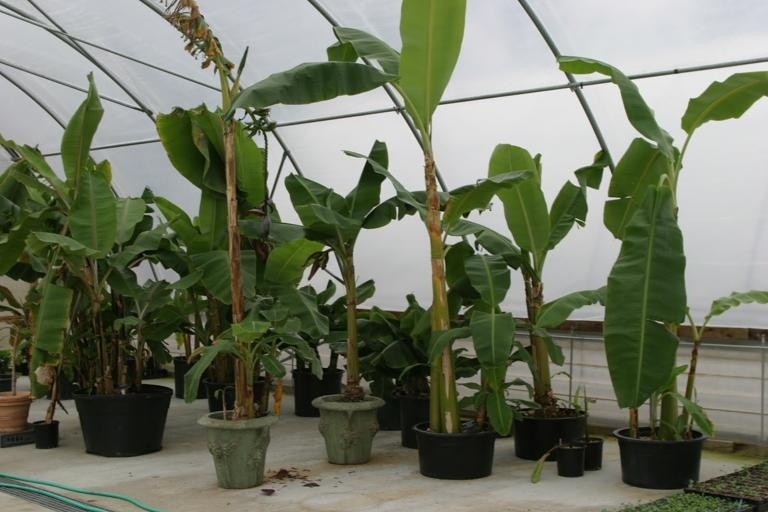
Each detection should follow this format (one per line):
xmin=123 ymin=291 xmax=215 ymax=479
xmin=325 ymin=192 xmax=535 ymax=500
xmin=0 ymin=1 xmax=768 ymax=490
